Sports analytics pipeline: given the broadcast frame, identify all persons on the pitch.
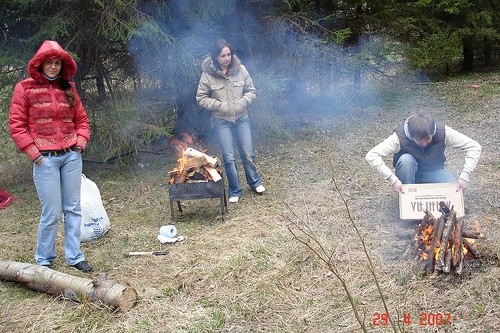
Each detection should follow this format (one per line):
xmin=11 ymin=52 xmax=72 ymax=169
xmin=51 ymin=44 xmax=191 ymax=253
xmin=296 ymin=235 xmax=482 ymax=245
xmin=195 ymin=38 xmax=267 ymax=204
xmin=8 ymin=38 xmax=93 ymax=273
xmin=364 ymin=113 xmax=482 ymax=201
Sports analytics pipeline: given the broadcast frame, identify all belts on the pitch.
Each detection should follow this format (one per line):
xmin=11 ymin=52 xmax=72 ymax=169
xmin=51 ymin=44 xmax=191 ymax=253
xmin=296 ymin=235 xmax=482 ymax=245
xmin=42 ymin=146 xmax=74 ymax=157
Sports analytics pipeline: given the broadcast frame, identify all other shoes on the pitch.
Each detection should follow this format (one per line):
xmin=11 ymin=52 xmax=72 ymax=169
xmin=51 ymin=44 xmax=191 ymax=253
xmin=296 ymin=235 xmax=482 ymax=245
xmin=74 ymin=261 xmax=90 ymax=271
xmin=229 ymin=197 xmax=239 ymax=203
xmin=256 ymin=185 xmax=266 ymax=193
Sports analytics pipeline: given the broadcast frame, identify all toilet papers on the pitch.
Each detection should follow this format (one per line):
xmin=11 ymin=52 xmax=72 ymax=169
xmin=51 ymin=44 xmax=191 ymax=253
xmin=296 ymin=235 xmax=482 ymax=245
xmin=157 ymin=225 xmax=184 ymax=244
